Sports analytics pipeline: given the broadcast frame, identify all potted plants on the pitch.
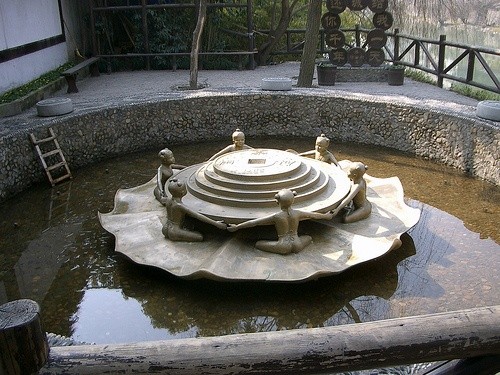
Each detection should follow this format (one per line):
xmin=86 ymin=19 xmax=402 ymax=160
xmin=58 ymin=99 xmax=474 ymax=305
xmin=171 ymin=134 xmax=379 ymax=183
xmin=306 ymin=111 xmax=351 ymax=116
xmin=385 ymin=64 xmax=405 ymax=87
xmin=316 ymin=61 xmax=338 ymax=86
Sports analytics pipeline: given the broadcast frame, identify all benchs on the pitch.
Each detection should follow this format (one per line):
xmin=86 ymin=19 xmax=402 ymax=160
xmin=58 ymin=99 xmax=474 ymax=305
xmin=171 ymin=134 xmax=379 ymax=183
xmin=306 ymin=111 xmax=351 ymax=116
xmin=59 ymin=56 xmax=101 ymax=94
xmin=126 ymin=51 xmax=254 ymax=72
xmin=100 ymin=53 xmax=183 ymax=74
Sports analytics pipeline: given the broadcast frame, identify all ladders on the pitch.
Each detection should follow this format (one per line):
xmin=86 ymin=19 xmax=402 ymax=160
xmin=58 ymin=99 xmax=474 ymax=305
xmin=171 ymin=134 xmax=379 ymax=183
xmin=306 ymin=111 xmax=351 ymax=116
xmin=30 ymin=126 xmax=72 ymax=187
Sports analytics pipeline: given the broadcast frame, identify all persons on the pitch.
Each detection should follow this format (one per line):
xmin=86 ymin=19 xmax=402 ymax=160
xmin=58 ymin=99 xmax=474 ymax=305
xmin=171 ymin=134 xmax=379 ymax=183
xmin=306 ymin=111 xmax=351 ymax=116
xmin=330 ymin=161 xmax=373 ymax=224
xmin=154 ymin=147 xmax=187 ymax=206
xmin=161 ymin=178 xmax=228 ymax=242
xmin=296 ymin=133 xmax=340 ymax=168
xmin=206 ymin=128 xmax=255 ymax=162
xmin=226 ymin=187 xmax=334 ymax=256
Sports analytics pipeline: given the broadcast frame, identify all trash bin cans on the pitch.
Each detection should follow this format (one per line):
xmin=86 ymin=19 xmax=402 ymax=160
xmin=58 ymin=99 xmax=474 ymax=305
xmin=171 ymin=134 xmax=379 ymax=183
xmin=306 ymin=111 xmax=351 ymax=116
xmin=316 ymin=64 xmax=338 ymax=86
